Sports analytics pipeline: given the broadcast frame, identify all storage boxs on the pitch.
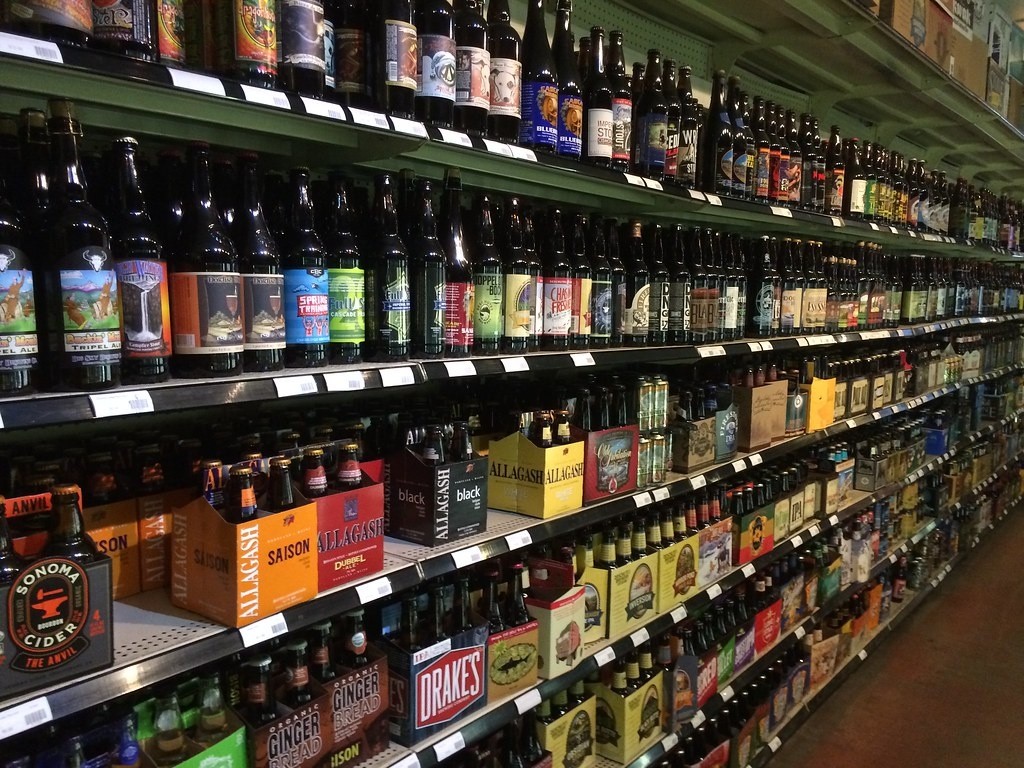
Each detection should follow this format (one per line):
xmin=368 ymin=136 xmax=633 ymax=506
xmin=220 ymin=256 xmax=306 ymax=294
xmin=0 ymin=378 xmax=1024 ymax=768
xmin=861 ymin=0 xmax=1024 ymax=133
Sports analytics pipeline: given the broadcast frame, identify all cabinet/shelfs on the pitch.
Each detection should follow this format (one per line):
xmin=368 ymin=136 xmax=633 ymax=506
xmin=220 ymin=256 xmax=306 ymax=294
xmin=0 ymin=0 xmax=1024 ymax=768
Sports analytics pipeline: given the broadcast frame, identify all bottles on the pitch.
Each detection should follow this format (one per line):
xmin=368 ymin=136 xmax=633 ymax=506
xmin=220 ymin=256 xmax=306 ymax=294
xmin=0 ymin=0 xmax=1024 ymax=768
